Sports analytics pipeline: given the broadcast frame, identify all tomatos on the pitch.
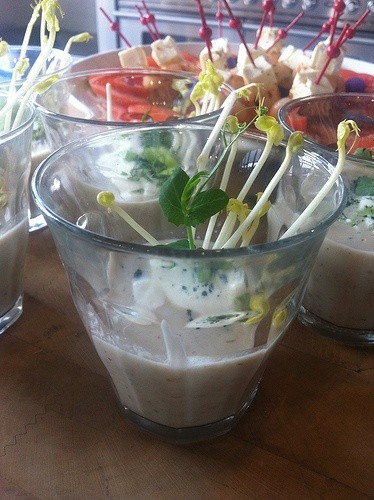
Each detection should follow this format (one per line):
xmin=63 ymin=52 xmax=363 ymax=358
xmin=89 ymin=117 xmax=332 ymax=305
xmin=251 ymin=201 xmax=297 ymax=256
xmin=104 ymin=101 xmax=184 ymax=123
xmin=90 ymin=69 xmax=175 ymax=108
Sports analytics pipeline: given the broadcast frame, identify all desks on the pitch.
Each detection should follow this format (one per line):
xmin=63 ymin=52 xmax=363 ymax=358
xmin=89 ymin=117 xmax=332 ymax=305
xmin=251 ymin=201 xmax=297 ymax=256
xmin=0 ymin=53 xmax=374 ymax=500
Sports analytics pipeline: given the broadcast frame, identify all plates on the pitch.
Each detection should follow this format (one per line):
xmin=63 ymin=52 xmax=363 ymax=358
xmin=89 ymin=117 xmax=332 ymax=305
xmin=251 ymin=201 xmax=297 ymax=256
xmin=65 ymin=41 xmax=374 ymax=112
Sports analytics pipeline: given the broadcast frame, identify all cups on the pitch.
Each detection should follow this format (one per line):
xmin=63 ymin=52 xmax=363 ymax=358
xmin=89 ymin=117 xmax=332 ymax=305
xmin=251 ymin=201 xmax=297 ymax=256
xmin=29 ymin=67 xmax=236 ymax=218
xmin=29 ymin=123 xmax=349 ymax=446
xmin=277 ymin=92 xmax=374 ymax=348
xmin=0 ymin=93 xmax=38 ymax=335
xmin=0 ymin=45 xmax=72 ymax=235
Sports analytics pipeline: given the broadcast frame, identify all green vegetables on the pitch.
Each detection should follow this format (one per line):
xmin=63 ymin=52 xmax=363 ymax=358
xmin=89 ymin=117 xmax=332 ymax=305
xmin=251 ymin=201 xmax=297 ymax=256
xmin=160 ymin=168 xmax=230 ymax=281
